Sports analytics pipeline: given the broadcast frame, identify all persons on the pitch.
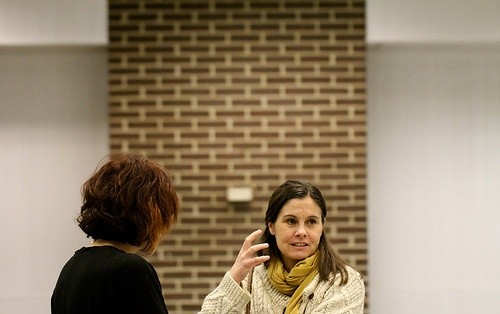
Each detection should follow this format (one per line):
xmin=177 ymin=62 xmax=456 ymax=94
xmin=51 ymin=153 xmax=180 ymax=314
xmin=197 ymin=180 xmax=365 ymax=314
